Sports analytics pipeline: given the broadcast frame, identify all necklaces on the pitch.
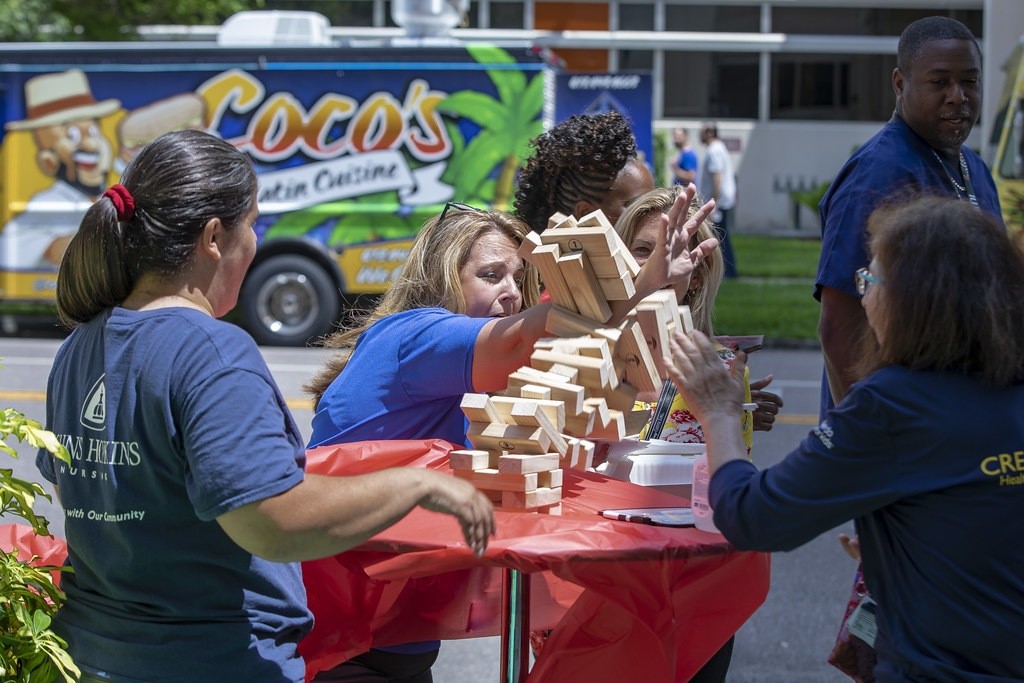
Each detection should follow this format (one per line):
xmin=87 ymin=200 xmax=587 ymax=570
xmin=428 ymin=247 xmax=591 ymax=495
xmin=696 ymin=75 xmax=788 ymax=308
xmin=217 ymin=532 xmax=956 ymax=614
xmin=951 ymin=177 xmax=966 ymax=192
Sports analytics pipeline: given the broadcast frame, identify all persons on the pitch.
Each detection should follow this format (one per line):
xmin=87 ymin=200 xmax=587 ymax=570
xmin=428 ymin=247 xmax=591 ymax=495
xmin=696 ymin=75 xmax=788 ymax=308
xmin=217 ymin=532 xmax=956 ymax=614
xmin=609 ymin=184 xmax=784 ymax=681
xmin=307 ymin=179 xmax=719 ymax=682
xmin=36 ymin=126 xmax=499 ymax=683
xmin=664 ymin=195 xmax=1024 ymax=683
xmin=811 ymin=15 xmax=1023 ymax=562
xmin=667 ymin=127 xmax=698 ymax=189
xmin=698 ymin=126 xmax=742 ymax=279
xmin=505 ymin=113 xmax=654 ymax=224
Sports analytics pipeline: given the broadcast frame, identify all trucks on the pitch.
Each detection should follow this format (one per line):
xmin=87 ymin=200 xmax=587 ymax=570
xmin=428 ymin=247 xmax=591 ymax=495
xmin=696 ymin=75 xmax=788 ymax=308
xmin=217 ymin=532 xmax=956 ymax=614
xmin=2 ymin=11 xmax=648 ymax=343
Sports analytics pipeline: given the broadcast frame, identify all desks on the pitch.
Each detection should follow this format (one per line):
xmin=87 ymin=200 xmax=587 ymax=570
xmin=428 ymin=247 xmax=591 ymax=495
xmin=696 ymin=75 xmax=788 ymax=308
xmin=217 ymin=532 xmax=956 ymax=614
xmin=304 ymin=446 xmax=737 ymax=683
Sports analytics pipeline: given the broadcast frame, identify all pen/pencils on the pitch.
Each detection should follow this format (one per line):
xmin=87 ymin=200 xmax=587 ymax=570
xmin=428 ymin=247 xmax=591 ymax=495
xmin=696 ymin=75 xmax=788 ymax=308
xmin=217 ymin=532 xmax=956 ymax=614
xmin=598 ymin=510 xmax=652 ymax=524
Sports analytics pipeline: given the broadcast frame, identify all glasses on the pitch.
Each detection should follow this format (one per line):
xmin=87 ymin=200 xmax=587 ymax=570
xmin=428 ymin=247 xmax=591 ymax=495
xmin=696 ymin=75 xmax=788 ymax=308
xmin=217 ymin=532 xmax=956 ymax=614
xmin=436 ymin=199 xmax=479 ymax=222
xmin=854 ymin=265 xmax=886 ymax=292
